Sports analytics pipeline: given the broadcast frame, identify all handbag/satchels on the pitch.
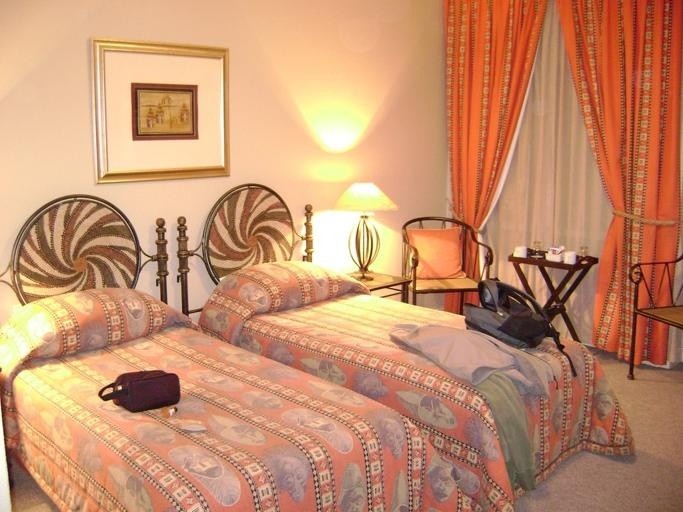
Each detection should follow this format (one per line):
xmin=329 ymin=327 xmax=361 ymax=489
xmin=98 ymin=370 xmax=180 ymax=412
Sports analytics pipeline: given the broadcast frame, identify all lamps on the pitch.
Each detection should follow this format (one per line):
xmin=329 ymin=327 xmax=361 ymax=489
xmin=338 ymin=182 xmax=398 ymax=282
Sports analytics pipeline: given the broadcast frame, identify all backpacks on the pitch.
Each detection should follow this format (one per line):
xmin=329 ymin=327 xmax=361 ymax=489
xmin=463 ymin=277 xmax=566 ymax=348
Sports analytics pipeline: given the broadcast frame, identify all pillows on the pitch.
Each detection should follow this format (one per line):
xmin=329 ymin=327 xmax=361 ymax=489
xmin=406 ymin=227 xmax=467 ymax=280
xmin=200 ymin=261 xmax=369 ymax=346
xmin=1 ymin=288 xmax=201 ymax=449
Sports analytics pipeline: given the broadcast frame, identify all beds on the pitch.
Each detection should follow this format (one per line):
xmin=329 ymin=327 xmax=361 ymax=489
xmin=177 ymin=183 xmax=636 ymax=511
xmin=1 ymin=195 xmax=482 ymax=511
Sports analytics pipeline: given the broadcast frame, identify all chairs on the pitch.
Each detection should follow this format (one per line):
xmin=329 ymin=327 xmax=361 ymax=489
xmin=627 ymin=252 xmax=683 ymax=379
xmin=401 ymin=216 xmax=494 ymax=315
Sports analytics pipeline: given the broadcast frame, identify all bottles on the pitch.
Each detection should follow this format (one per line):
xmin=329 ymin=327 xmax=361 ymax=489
xmin=486 ymin=254 xmax=496 ymax=280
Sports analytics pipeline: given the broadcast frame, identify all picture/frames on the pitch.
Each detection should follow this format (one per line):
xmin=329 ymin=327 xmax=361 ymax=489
xmin=90 ymin=38 xmax=231 ymax=183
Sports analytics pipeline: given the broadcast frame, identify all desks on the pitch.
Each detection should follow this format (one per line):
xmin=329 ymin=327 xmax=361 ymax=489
xmin=508 ymin=252 xmax=599 ymax=342
xmin=348 ymin=272 xmax=412 ymax=303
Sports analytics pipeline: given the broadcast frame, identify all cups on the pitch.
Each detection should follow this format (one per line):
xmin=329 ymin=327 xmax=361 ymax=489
xmin=513 ymin=246 xmax=527 ymax=257
xmin=532 ymin=242 xmax=543 ymax=256
xmin=579 ymin=246 xmax=588 ymax=264
xmin=561 ymin=251 xmax=576 ymax=265
